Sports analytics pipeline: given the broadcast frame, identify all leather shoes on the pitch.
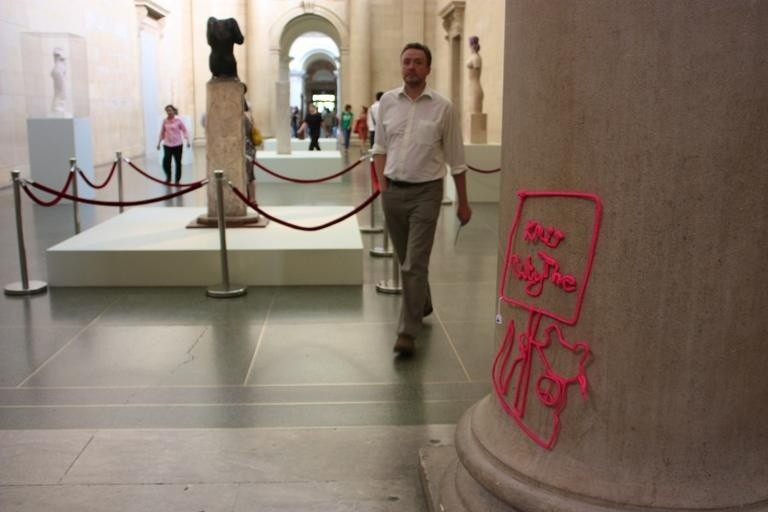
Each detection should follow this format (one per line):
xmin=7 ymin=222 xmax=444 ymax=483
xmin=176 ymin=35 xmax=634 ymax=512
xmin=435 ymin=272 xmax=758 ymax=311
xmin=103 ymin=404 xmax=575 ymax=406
xmin=423 ymin=304 xmax=433 ymax=317
xmin=392 ymin=332 xmax=416 ymax=355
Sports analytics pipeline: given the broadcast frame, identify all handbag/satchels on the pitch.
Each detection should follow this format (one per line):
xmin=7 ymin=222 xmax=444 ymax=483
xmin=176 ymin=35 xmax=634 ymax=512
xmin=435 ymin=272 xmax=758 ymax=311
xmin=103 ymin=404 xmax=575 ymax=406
xmin=251 ymin=123 xmax=262 ymax=145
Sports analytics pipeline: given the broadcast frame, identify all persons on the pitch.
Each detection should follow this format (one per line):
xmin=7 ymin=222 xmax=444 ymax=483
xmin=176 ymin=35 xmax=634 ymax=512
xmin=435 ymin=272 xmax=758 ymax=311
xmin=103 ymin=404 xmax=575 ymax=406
xmin=374 ymin=42 xmax=473 ymax=353
xmin=243 ymin=84 xmax=259 ymax=211
xmin=157 ymin=104 xmax=190 ymax=186
xmin=49 ymin=47 xmax=69 ymax=115
xmin=466 ymin=34 xmax=484 ymax=111
xmin=207 ymin=17 xmax=243 ymax=76
xmin=289 ymin=92 xmax=385 ymax=154
